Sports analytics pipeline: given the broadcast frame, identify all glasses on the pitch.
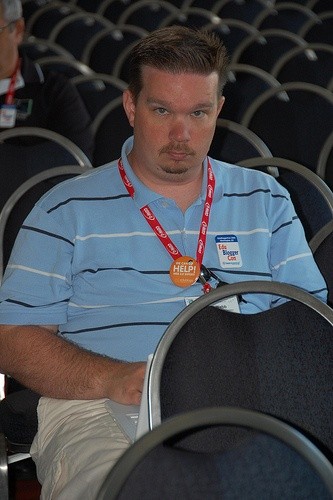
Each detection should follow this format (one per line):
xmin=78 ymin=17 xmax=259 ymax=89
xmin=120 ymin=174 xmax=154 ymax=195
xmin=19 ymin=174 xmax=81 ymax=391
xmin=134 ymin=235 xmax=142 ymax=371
xmin=0 ymin=21 xmax=15 ymax=33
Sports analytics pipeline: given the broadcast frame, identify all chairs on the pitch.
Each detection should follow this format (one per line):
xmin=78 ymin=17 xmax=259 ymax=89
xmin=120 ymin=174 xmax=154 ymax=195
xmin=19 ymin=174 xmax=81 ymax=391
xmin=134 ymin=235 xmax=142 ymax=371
xmin=0 ymin=0 xmax=333 ymax=500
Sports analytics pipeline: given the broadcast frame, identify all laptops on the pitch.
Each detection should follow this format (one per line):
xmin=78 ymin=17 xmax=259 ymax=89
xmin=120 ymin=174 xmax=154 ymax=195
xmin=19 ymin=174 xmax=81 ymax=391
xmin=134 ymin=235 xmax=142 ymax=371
xmin=105 ymin=354 xmax=154 ymax=445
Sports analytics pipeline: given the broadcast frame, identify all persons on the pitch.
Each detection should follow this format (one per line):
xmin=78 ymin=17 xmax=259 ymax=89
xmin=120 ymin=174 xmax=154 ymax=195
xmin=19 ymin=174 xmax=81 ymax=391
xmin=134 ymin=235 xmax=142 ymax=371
xmin=0 ymin=0 xmax=95 ymax=161
xmin=0 ymin=27 xmax=327 ymax=499
